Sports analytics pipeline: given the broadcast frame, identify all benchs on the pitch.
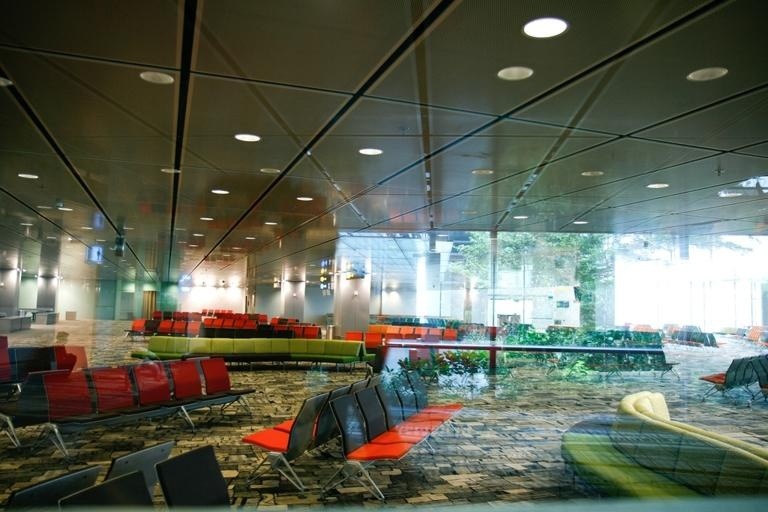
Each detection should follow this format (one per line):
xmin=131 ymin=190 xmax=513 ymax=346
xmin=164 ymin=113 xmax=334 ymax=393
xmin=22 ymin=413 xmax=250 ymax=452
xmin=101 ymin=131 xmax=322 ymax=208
xmin=556 ymin=389 xmax=768 ymax=511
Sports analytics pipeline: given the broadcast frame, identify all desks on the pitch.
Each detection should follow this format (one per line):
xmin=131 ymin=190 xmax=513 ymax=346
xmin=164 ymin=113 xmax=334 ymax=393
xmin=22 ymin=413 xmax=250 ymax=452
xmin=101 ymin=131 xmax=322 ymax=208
xmin=0 ymin=306 xmax=57 ymax=334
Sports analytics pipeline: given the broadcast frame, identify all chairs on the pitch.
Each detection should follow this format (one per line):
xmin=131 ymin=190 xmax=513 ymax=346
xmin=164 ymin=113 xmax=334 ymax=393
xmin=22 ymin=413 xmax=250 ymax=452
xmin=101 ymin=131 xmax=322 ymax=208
xmin=242 ymin=367 xmax=463 ymax=502
xmin=101 ymin=442 xmax=174 ymax=501
xmin=546 ymin=320 xmax=767 ymax=350
xmin=4 ymin=342 xmax=259 ymax=457
xmin=127 ymin=306 xmax=498 ymax=372
xmin=155 ymin=442 xmax=233 ymax=510
xmin=4 ymin=463 xmax=97 ymax=509
xmin=698 ymin=354 xmax=768 ymax=408
xmin=58 ymin=471 xmax=157 ymax=510
